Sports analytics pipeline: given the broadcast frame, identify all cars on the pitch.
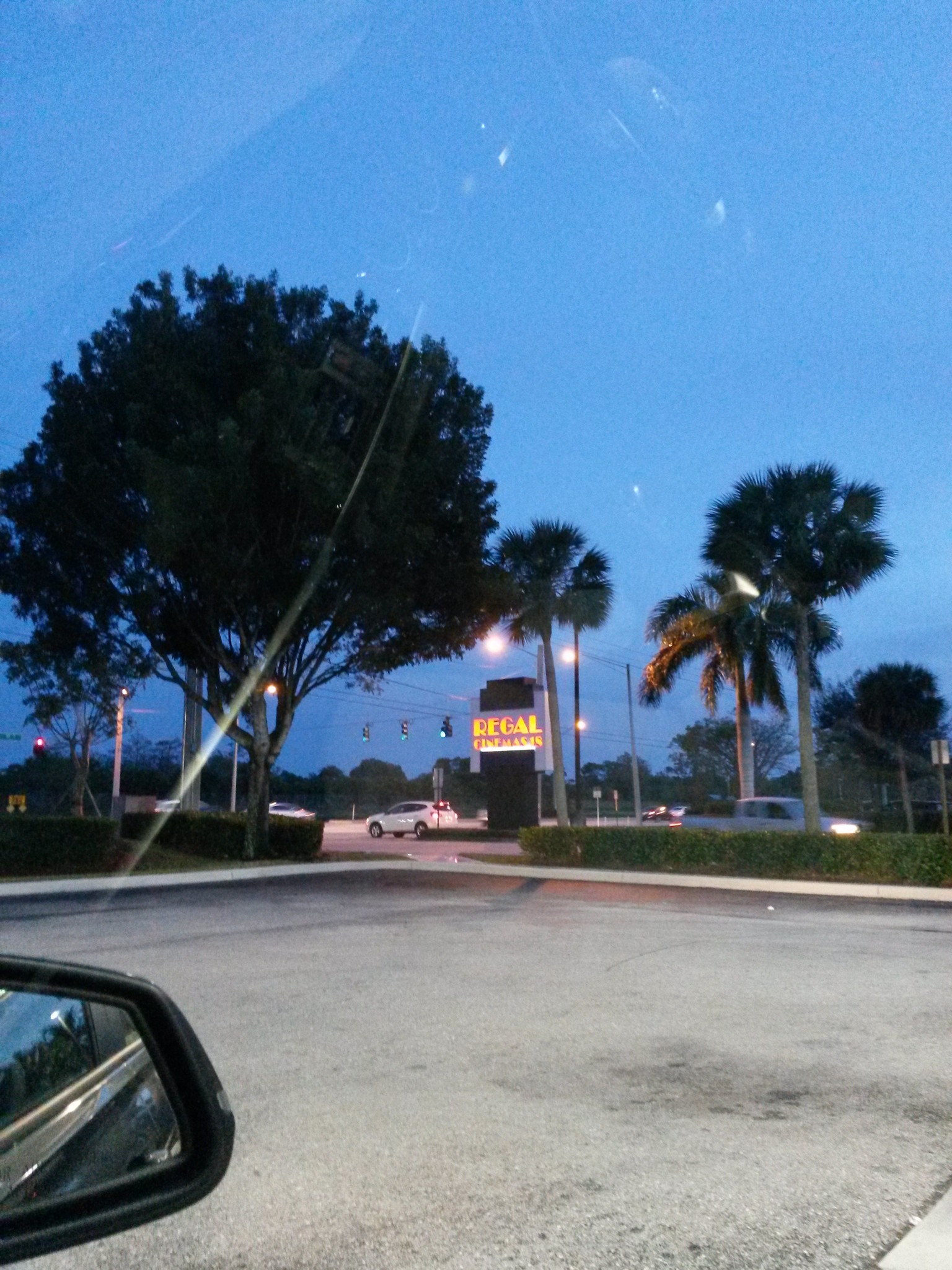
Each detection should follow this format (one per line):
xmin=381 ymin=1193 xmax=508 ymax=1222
xmin=154 ymin=798 xmax=213 ymax=814
xmin=641 ymin=805 xmax=669 ymax=822
xmin=667 ymin=806 xmax=691 ymax=819
xmin=242 ymin=802 xmax=318 ymax=822
xmin=364 ymin=800 xmax=459 ymax=840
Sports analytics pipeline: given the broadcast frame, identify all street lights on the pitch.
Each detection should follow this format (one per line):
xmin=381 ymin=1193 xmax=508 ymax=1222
xmin=50 ymin=1009 xmax=93 ymax=1069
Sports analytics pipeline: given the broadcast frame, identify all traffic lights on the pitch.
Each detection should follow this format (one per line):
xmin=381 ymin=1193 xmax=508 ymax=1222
xmin=33 ymin=738 xmax=44 ymax=755
xmin=440 ymin=720 xmax=452 ymax=738
xmin=401 ymin=722 xmax=408 ymax=740
xmin=363 ymin=727 xmax=370 ymax=742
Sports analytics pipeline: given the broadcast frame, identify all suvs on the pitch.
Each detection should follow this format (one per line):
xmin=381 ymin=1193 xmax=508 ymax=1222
xmin=732 ymin=796 xmax=876 ymax=835
